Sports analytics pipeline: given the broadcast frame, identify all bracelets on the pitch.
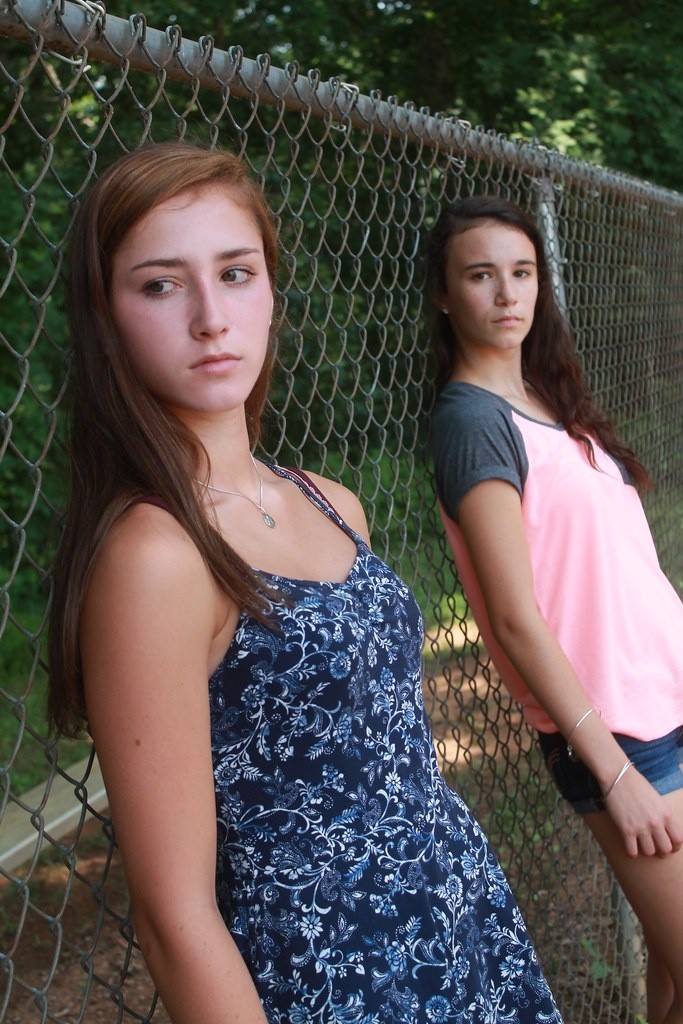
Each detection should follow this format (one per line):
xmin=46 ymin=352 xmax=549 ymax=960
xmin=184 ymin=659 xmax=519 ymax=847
xmin=599 ymin=756 xmax=637 ymax=805
xmin=566 ymin=707 xmax=602 ymax=764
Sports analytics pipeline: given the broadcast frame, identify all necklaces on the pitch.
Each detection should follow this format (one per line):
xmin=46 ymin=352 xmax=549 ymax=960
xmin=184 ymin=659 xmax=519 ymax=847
xmin=191 ymin=452 xmax=276 ymax=530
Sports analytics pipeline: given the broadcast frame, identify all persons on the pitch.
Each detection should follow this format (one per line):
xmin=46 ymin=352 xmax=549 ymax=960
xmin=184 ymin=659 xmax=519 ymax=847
xmin=49 ymin=144 xmax=566 ymax=1024
xmin=420 ymin=197 xmax=683 ymax=1024
